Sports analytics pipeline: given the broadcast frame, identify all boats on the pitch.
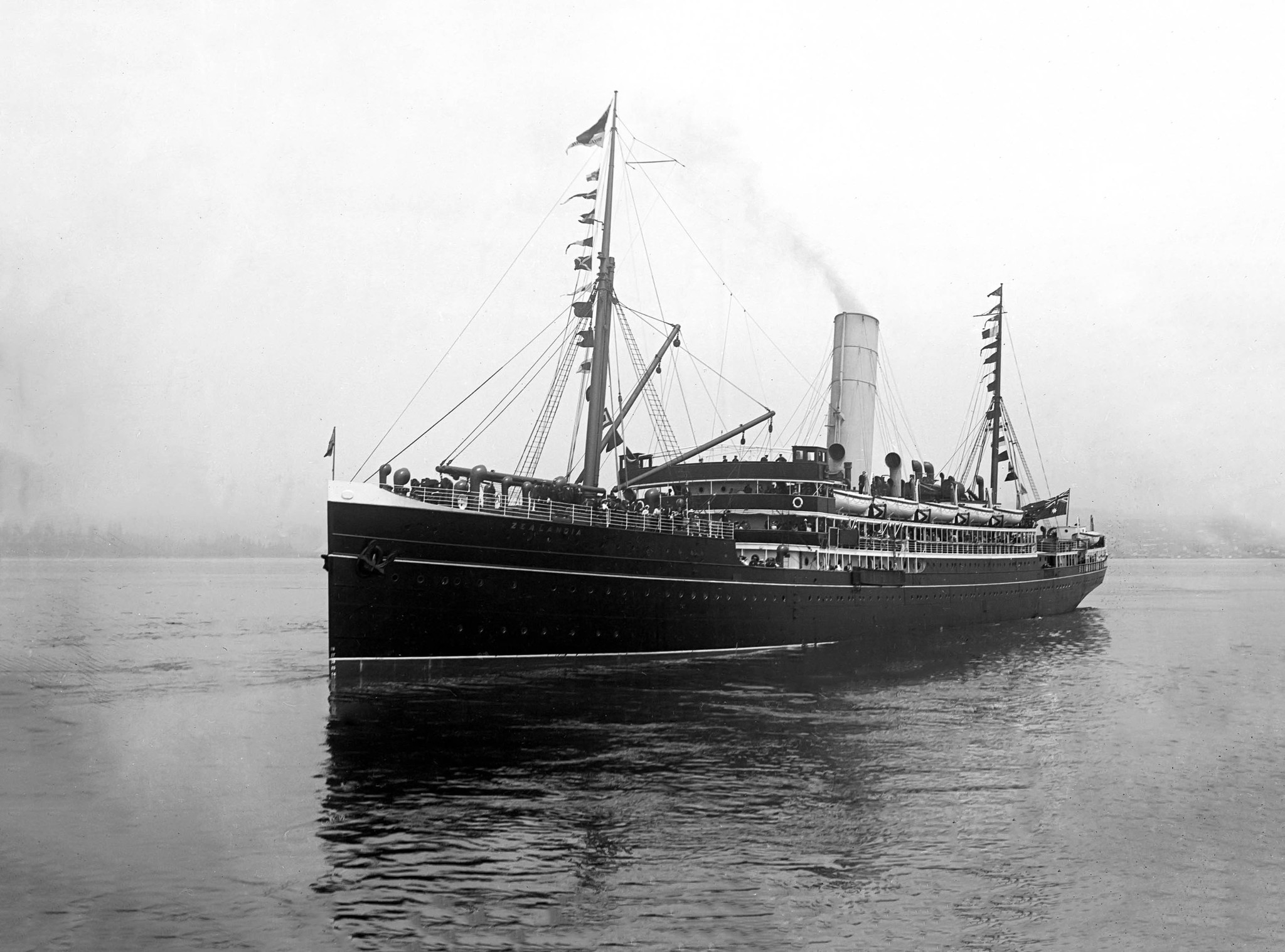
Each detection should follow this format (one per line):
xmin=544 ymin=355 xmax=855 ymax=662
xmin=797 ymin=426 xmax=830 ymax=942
xmin=321 ymin=86 xmax=1110 ymax=700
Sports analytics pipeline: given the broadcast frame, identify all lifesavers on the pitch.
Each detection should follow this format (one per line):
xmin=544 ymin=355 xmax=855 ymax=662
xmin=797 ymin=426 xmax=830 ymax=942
xmin=793 ymin=497 xmax=803 ymax=508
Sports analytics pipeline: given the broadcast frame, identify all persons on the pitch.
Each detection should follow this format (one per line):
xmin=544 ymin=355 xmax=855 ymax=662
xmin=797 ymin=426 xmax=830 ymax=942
xmin=722 ymin=456 xmax=727 ymax=462
xmin=776 ymin=454 xmax=787 ymax=462
xmin=732 ymin=455 xmax=739 ymax=461
xmin=858 ymin=471 xmax=866 ymax=494
xmin=760 ymin=454 xmax=768 ymax=462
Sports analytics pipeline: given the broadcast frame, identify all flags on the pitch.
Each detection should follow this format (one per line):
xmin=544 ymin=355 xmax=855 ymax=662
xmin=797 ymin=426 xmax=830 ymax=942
xmin=323 ymin=427 xmax=334 ymax=457
xmin=1022 ymin=488 xmax=1068 ymax=522
